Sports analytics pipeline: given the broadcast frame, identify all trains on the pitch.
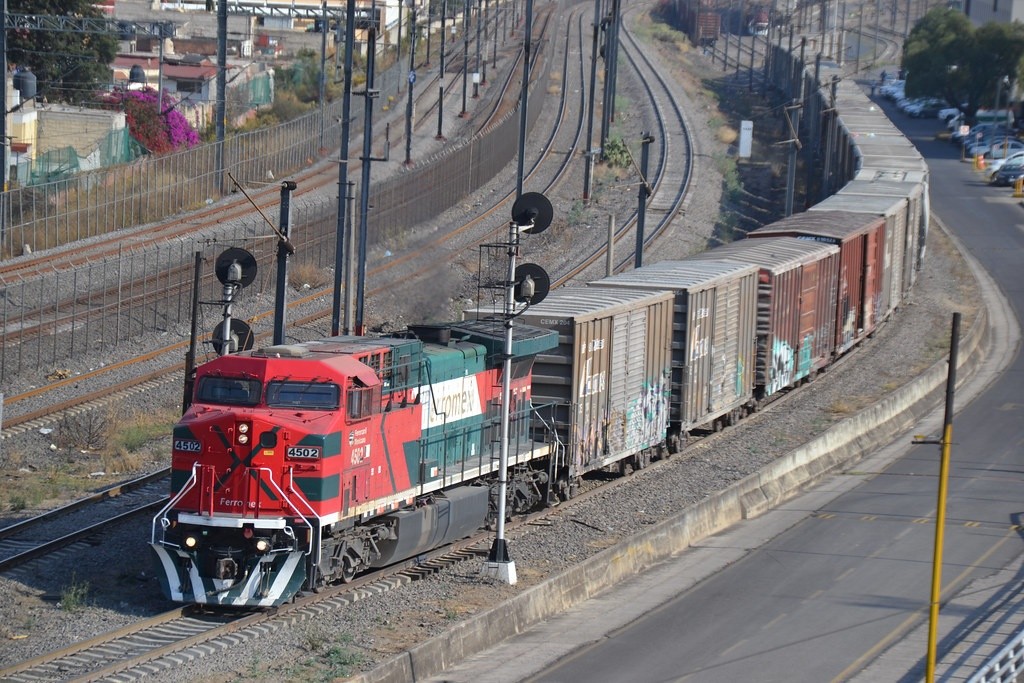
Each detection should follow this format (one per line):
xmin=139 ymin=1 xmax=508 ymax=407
xmin=662 ymin=0 xmax=722 ymax=46
xmin=149 ymin=39 xmax=931 ymax=618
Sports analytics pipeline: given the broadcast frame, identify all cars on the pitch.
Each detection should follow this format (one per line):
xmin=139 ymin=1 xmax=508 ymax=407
xmin=878 ymin=76 xmax=1024 ymax=190
